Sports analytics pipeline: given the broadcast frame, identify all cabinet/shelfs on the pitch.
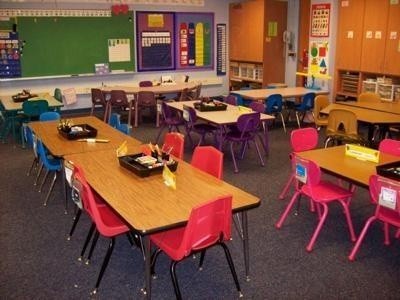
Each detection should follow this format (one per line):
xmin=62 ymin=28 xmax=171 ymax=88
xmin=334 ymin=1 xmax=364 ymax=106
xmin=229 ymin=1 xmax=286 ymax=90
xmin=361 ymin=0 xmax=389 ymax=76
xmin=384 ymin=0 xmax=400 ymax=76
xmin=361 ymin=75 xmax=399 ymax=106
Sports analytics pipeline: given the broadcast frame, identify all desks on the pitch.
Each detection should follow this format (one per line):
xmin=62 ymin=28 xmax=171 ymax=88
xmin=294 ymin=143 xmax=400 ymax=217
xmin=319 ymin=102 xmax=400 ymax=147
xmin=26 ymin=115 xmax=143 ymax=216
xmin=230 ymin=87 xmax=330 ymax=126
xmin=96 ymin=79 xmax=201 ymax=128
xmin=63 ymin=144 xmax=262 ymax=300
xmin=0 ymin=92 xmax=65 ymax=147
xmin=167 ymin=99 xmax=276 ymax=157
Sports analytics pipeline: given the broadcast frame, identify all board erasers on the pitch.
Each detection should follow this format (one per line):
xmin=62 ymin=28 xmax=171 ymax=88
xmin=72 ymin=75 xmax=78 ymax=76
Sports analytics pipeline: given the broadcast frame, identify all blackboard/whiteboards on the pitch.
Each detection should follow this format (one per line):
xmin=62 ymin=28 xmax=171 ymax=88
xmin=0 ymin=9 xmax=137 ymax=82
xmin=141 ymin=30 xmax=172 ymax=68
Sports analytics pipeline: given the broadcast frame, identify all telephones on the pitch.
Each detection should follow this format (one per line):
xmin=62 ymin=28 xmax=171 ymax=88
xmin=283 ymin=31 xmax=292 ymax=44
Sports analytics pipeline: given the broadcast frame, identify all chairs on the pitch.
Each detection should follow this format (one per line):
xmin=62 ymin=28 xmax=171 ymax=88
xmin=154 ymin=101 xmax=194 ymax=149
xmin=47 ymin=88 xmax=62 ymax=115
xmin=39 ymin=112 xmax=61 ymax=122
xmin=324 ymin=108 xmax=366 ymax=149
xmin=103 ymin=89 xmax=134 ymax=127
xmin=348 ymin=174 xmax=400 ymax=262
xmin=225 ymin=111 xmax=265 ymax=174
xmin=70 ymin=163 xmax=85 ymax=221
xmin=188 ymin=82 xmax=203 ymax=100
xmin=275 ymin=156 xmax=358 ymax=254
xmin=182 ymin=104 xmax=206 ymax=148
xmin=225 ymin=95 xmax=236 ymax=104
xmin=359 ymin=92 xmax=388 ymax=143
xmin=27 ymin=133 xmax=55 ymax=175
xmin=241 ymin=102 xmax=266 ymax=150
xmin=278 ymin=127 xmax=344 ymax=214
xmin=262 ymin=93 xmax=287 ymax=134
xmin=162 ymin=131 xmax=184 ymax=159
xmin=267 ymin=85 xmax=277 ymax=89
xmin=19 ymin=99 xmax=49 ymax=144
xmin=227 ymin=93 xmax=253 ymax=135
xmin=139 ymin=81 xmax=155 ymax=110
xmin=66 ymin=173 xmax=145 ymax=261
xmin=187 ymin=106 xmax=223 ymax=155
xmin=188 ymin=145 xmax=240 ymax=272
xmin=145 ymin=193 xmax=244 ymax=300
xmin=90 ymin=87 xmax=111 ymax=123
xmin=160 ymin=74 xmax=173 ymax=83
xmin=314 ymin=95 xmax=329 ymax=132
xmin=108 ymin=112 xmax=120 ymax=131
xmin=378 ymin=139 xmax=400 ymax=157
xmin=0 ymin=104 xmax=16 ymax=143
xmin=284 ymin=92 xmax=318 ymax=129
xmin=119 ymin=124 xmax=129 ymax=135
xmin=167 ymin=87 xmax=190 ymax=119
xmin=240 ymin=86 xmax=252 ymax=90
xmin=33 ymin=139 xmax=64 ymax=193
xmin=74 ymin=176 xmax=159 ymax=296
xmin=133 ymin=90 xmax=161 ymax=129
